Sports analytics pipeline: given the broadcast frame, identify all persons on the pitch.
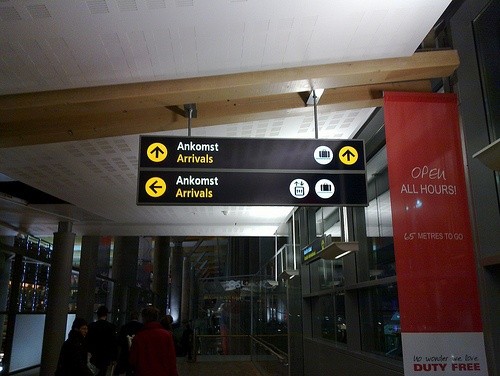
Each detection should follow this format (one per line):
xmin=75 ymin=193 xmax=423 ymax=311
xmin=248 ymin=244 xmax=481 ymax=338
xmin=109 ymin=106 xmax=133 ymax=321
xmin=55 ymin=318 xmax=92 ymax=376
xmin=129 ymin=307 xmax=180 ymax=376
xmin=85 ymin=306 xmax=118 ymax=376
xmin=115 ymin=311 xmax=146 ymax=376
xmin=182 ymin=324 xmax=193 ymax=355
